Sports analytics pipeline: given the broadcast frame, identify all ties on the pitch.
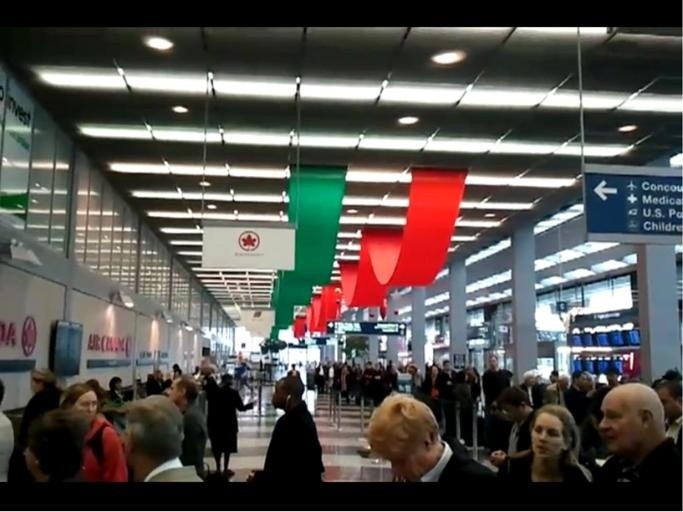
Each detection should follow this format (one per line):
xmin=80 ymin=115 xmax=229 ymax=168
xmin=508 ymin=425 xmax=520 ymax=455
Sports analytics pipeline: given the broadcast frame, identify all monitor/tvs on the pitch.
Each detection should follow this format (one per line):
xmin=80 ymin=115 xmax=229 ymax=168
xmin=50 ymin=319 xmax=83 ymax=377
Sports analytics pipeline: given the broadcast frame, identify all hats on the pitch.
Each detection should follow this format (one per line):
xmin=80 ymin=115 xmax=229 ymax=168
xmin=31 ymin=368 xmax=57 ymax=382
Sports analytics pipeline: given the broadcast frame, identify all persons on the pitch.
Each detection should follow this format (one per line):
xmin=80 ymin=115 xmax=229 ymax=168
xmin=288 ymin=356 xmax=682 ymax=462
xmin=246 ymin=376 xmax=325 ymax=482
xmin=24 ymin=407 xmax=91 ymax=481
xmin=497 ymin=404 xmax=593 ymax=482
xmin=19 ymin=357 xmax=259 ymax=476
xmin=489 ymin=385 xmax=537 ymax=483
xmin=123 ymin=395 xmax=204 ymax=482
xmin=0 ymin=379 xmax=15 ymax=482
xmin=59 ymin=383 xmax=129 ymax=482
xmin=599 ymin=383 xmax=676 ymax=485
xmin=368 ymin=390 xmax=496 ymax=482
